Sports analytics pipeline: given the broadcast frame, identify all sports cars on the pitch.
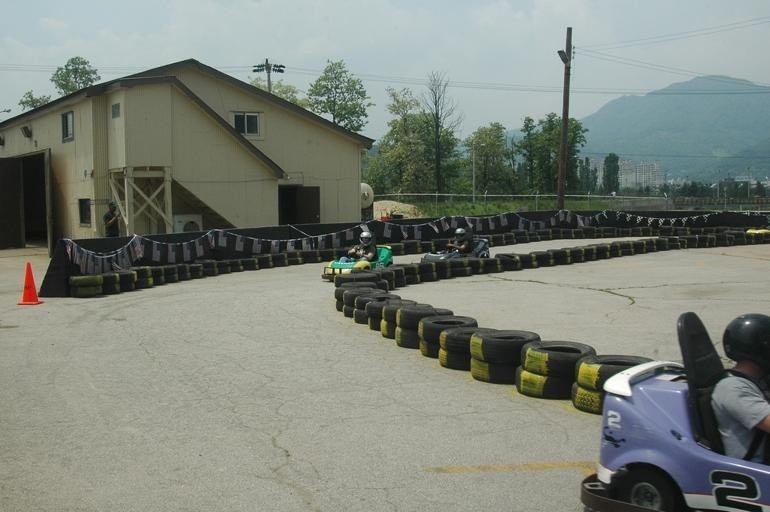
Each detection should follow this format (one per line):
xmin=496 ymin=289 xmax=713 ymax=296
xmin=581 ymin=313 xmax=770 ymax=512
xmin=322 ymin=245 xmax=393 ymax=281
xmin=745 ymin=224 xmax=770 ymax=236
xmin=421 ymin=239 xmax=489 ymax=263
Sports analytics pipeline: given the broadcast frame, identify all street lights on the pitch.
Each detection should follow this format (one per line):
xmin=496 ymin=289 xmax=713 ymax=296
xmin=557 ymin=27 xmax=572 ymax=209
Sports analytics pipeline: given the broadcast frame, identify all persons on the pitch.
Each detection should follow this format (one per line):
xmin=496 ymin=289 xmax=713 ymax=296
xmin=103 ymin=200 xmax=122 ymax=237
xmin=339 ymin=230 xmax=377 ymax=263
xmin=445 ymin=226 xmax=470 ymax=253
xmin=703 ymin=308 xmax=769 ymax=465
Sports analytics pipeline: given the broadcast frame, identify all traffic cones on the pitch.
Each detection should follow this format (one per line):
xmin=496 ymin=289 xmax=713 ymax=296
xmin=18 ymin=262 xmax=42 ymax=305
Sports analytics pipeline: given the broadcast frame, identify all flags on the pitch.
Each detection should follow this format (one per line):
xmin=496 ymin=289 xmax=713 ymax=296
xmin=383 ymin=210 xmax=608 ymax=242
xmin=346 ymin=229 xmax=353 ymax=240
xmin=219 ymin=231 xmax=279 ymax=254
xmin=152 ymin=229 xmax=214 ymax=263
xmin=360 ymin=224 xmax=368 ymax=231
xmin=287 ymin=233 xmax=338 ymax=252
xmin=65 ymin=237 xmax=144 ymax=276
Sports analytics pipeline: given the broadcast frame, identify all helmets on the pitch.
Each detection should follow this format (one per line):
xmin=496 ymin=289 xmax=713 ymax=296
xmin=453 ymin=227 xmax=467 ymax=242
xmin=722 ymin=309 xmax=770 ymax=364
xmin=358 ymin=231 xmax=372 ymax=246
